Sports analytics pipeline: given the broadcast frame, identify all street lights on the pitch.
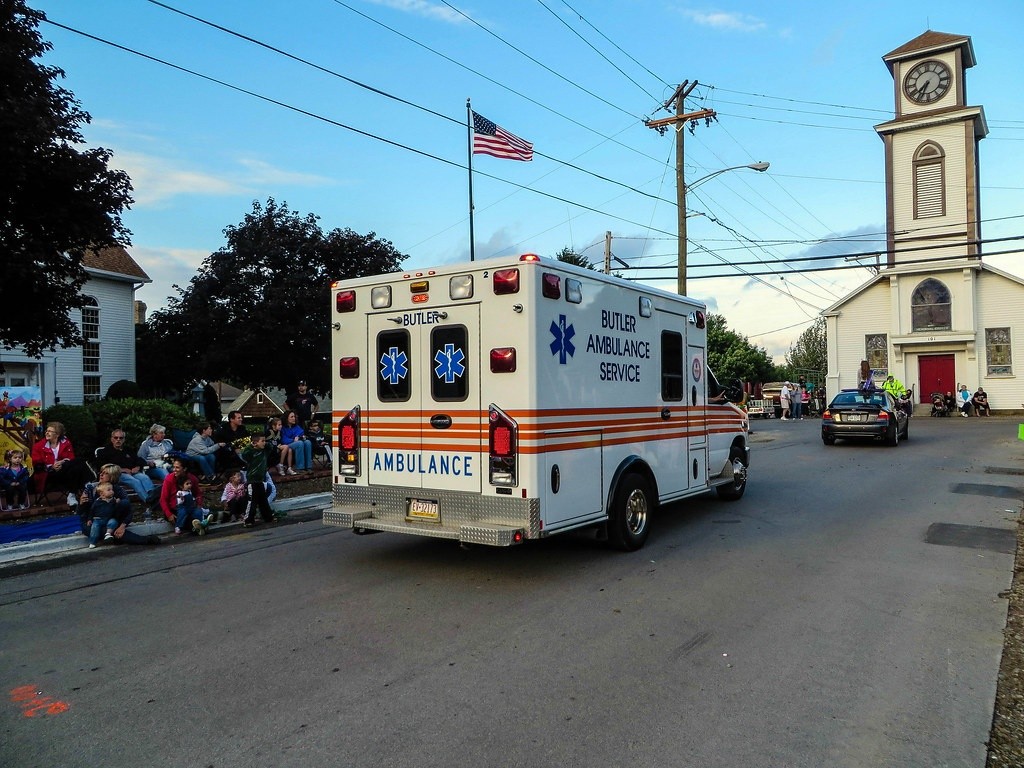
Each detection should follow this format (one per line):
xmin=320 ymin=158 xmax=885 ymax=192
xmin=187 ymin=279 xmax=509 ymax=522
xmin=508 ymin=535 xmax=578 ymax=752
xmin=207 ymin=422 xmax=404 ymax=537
xmin=678 ymin=160 xmax=770 ymax=297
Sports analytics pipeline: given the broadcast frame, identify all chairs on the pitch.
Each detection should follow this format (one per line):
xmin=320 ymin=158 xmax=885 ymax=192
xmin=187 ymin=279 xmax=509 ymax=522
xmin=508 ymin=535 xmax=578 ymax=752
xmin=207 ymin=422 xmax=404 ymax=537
xmin=304 ymin=420 xmax=326 ymax=471
xmin=0 ymin=483 xmax=70 ymax=512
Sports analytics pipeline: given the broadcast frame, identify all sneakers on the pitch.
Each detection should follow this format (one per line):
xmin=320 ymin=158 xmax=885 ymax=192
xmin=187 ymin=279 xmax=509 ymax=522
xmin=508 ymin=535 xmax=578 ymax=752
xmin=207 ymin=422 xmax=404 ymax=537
xmin=66 ymin=493 xmax=79 ymax=506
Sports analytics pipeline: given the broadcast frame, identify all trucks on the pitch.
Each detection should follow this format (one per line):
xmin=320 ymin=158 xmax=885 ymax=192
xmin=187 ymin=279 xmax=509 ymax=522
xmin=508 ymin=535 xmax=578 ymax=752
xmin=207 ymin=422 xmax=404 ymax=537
xmin=761 ymin=381 xmax=801 ymax=417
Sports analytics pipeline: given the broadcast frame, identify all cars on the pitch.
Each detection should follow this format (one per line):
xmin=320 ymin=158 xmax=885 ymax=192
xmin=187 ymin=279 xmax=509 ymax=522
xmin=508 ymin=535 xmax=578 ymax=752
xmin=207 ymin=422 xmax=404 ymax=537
xmin=820 ymin=387 xmax=910 ymax=448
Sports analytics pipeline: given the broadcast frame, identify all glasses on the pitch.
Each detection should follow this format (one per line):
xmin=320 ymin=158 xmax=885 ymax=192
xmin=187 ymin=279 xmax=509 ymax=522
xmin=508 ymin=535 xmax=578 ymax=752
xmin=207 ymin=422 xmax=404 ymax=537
xmin=45 ymin=430 xmax=54 ymax=433
xmin=114 ymin=437 xmax=124 ymax=439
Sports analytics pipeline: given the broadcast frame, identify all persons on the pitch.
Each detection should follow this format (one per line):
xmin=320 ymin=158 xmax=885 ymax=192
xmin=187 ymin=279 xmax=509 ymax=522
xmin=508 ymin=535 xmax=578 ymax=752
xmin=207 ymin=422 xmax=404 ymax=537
xmin=857 ymin=361 xmax=914 ymax=419
xmin=956 ymin=385 xmax=973 ymax=417
xmin=780 ymin=376 xmax=826 ymax=421
xmin=972 ymin=387 xmax=990 ymax=417
xmin=0 ymin=381 xmax=331 ymax=553
xmin=708 ymin=390 xmax=727 ymax=404
xmin=943 ymin=392 xmax=955 ymax=413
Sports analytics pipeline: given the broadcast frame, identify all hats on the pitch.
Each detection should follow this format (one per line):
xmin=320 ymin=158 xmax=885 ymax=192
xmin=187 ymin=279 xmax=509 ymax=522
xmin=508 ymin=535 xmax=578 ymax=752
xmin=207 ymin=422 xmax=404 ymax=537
xmin=786 ymin=381 xmax=790 ymax=385
xmin=298 ymin=379 xmax=306 ymax=386
xmin=978 ymin=387 xmax=983 ymax=391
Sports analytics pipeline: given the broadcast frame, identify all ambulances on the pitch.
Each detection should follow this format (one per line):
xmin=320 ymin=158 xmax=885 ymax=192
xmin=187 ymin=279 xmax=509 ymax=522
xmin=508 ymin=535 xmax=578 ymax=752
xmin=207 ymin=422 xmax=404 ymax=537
xmin=321 ymin=253 xmax=749 ymax=552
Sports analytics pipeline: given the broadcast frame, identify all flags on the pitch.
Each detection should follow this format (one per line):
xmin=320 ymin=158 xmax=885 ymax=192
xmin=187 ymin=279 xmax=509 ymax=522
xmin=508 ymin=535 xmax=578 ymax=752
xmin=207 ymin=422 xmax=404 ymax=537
xmin=470 ymin=108 xmax=533 ymax=163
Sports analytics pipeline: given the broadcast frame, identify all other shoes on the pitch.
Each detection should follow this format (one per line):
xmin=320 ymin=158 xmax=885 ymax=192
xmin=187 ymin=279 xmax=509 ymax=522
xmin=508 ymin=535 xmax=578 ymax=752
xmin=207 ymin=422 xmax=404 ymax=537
xmin=961 ymin=412 xmax=968 ymax=417
xmin=230 ymin=514 xmax=237 ymax=522
xmin=265 ymin=520 xmax=283 ymax=528
xmin=294 ymin=469 xmax=307 ymax=474
xmin=198 ymin=474 xmax=205 ymax=480
xmin=205 ymin=513 xmax=213 ymax=526
xmin=246 ymin=523 xmax=254 ymax=528
xmin=103 ymin=533 xmax=114 ymax=543
xmin=780 ymin=416 xmax=789 ymax=420
xmin=238 ymin=513 xmax=248 ymax=521
xmin=175 ymin=527 xmax=183 ymax=537
xmin=192 ymin=519 xmax=206 ymax=536
xmin=286 ymin=467 xmax=297 ymax=476
xmin=275 ymin=463 xmax=285 ymax=476
xmin=147 ymin=535 xmax=161 ymax=545
xmin=7 ymin=504 xmax=13 ymax=510
xmin=88 ymin=544 xmax=96 ymax=549
xmin=146 ymin=486 xmax=162 ymax=510
xmin=209 ymin=474 xmax=217 ymax=485
xmin=19 ymin=504 xmax=26 ymax=509
xmin=306 ymin=469 xmax=314 ymax=474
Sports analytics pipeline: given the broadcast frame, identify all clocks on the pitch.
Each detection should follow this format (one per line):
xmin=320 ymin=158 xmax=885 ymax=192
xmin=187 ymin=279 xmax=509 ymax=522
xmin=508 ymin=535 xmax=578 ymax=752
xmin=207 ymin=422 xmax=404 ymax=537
xmin=902 ymin=58 xmax=953 ymax=106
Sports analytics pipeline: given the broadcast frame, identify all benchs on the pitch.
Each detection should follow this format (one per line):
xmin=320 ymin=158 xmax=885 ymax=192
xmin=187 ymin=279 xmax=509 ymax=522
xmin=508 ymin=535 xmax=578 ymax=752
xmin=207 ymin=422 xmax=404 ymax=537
xmin=86 ymin=447 xmax=163 ymax=505
